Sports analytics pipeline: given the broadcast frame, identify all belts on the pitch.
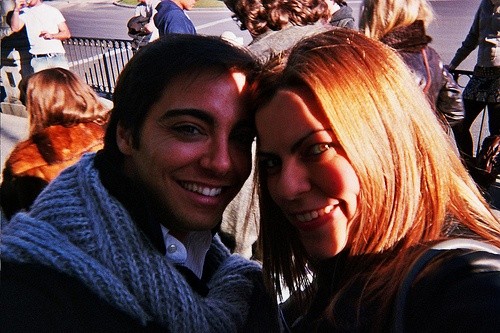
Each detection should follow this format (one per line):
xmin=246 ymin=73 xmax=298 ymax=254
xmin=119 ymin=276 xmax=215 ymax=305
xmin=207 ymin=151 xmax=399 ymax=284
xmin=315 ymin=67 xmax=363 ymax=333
xmin=32 ymin=53 xmax=57 ymax=58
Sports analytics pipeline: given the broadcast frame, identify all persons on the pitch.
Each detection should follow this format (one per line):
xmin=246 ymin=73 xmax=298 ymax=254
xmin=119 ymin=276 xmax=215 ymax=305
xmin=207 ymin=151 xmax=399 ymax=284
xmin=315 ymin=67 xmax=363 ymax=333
xmin=358 ymin=0 xmax=465 ymax=128
xmin=223 ymin=0 xmax=356 ymax=63
xmin=134 ymin=0 xmax=197 ymax=42
xmin=11 ymin=0 xmax=71 ymax=73
xmin=0 ymin=34 xmax=283 ymax=333
xmin=0 ymin=67 xmax=110 ymax=222
xmin=247 ymin=29 xmax=500 ymax=333
xmin=446 ymin=0 xmax=500 ymax=159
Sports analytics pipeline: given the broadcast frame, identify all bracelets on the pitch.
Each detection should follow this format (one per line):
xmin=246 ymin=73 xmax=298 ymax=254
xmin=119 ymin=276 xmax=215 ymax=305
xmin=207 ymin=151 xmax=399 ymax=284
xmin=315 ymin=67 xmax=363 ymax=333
xmin=52 ymin=34 xmax=54 ymax=39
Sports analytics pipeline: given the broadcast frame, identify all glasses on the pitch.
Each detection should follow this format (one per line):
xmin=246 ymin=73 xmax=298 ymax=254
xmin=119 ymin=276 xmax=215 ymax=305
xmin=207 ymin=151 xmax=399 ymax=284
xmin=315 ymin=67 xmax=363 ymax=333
xmin=232 ymin=14 xmax=243 ymax=28
